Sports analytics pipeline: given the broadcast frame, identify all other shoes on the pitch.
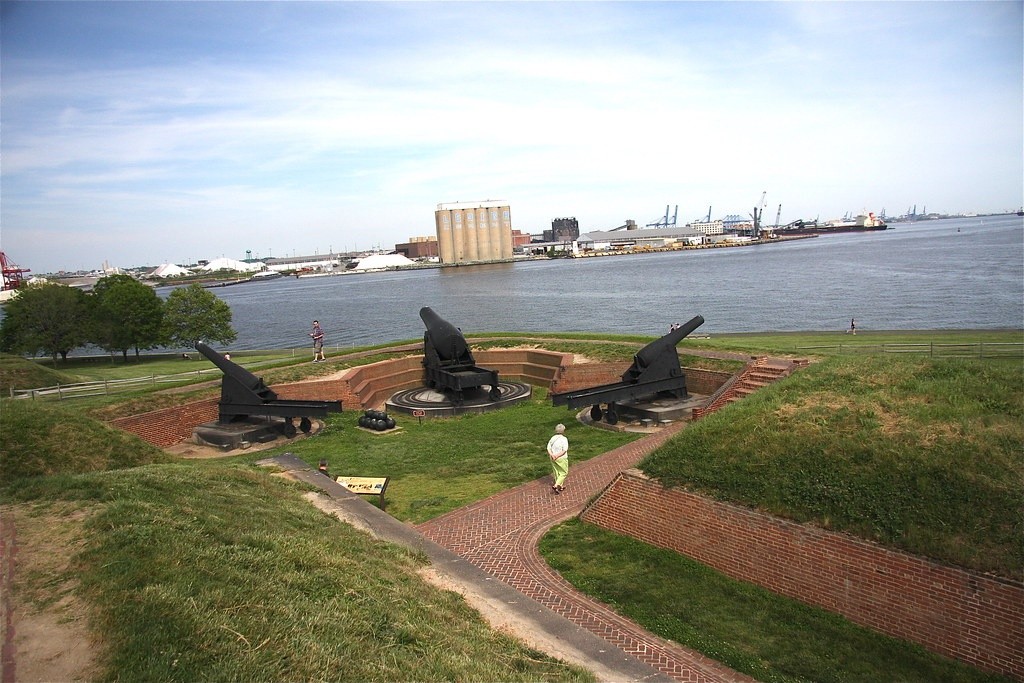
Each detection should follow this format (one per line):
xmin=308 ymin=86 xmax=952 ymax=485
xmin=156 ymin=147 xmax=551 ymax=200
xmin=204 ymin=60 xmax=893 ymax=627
xmin=319 ymin=359 xmax=325 ymax=362
xmin=552 ymin=485 xmax=559 ymax=495
xmin=559 ymin=486 xmax=566 ymax=491
xmin=312 ymin=360 xmax=317 ymax=363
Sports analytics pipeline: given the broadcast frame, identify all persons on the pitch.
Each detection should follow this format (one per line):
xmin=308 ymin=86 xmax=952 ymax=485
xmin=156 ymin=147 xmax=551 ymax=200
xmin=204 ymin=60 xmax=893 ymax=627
xmin=225 ymin=352 xmax=230 ymax=360
xmin=669 ymin=323 xmax=677 ymax=333
xmin=310 ymin=320 xmax=325 ymax=362
xmin=547 ymin=424 xmax=568 ymax=495
xmin=318 ymin=458 xmax=330 ymax=478
xmin=184 ymin=353 xmax=192 ymax=359
xmin=847 ymin=318 xmax=856 ymax=335
xmin=676 ymin=323 xmax=680 ymax=329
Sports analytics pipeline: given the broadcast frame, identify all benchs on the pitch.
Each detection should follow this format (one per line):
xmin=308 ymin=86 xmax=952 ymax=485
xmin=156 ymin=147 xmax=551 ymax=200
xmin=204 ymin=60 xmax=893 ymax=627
xmin=686 ymin=334 xmax=709 ymax=340
